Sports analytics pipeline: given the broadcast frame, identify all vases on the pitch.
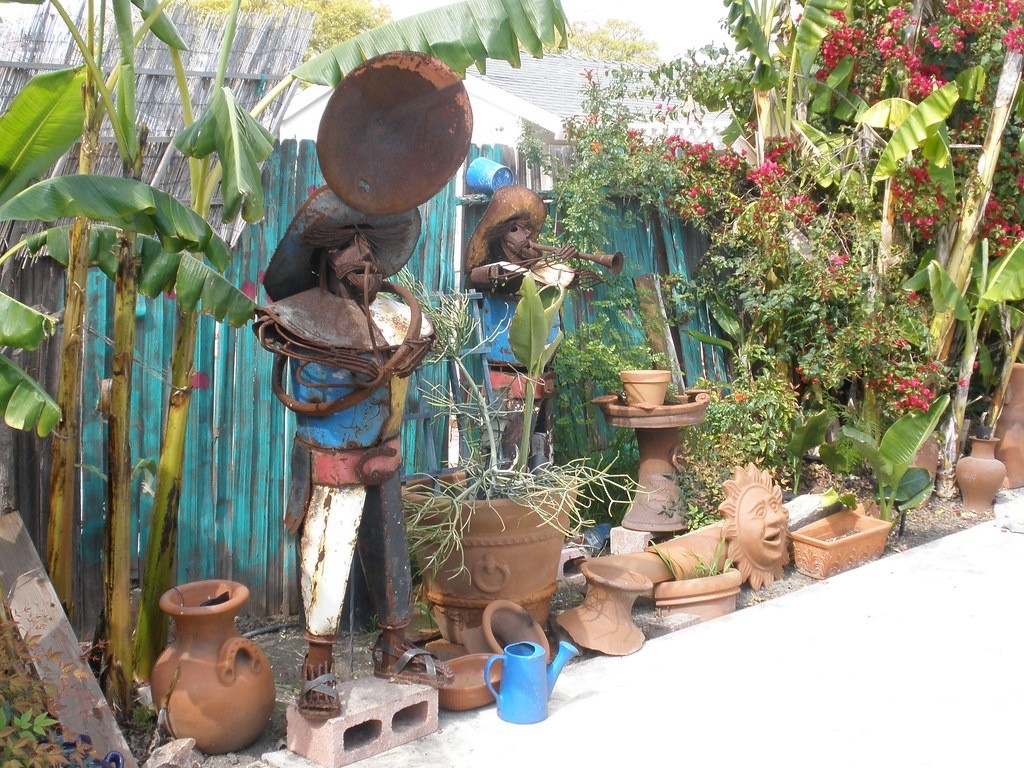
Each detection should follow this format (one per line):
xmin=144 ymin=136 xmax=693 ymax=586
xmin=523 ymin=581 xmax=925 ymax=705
xmin=149 ymin=369 xmax=1024 ymax=757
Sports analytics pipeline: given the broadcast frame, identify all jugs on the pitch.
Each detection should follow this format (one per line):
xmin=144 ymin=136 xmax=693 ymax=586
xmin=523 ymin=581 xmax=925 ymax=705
xmin=483 ymin=639 xmax=579 ymax=725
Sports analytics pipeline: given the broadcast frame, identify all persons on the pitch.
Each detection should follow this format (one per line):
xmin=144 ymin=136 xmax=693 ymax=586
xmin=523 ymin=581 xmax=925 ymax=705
xmin=250 ymin=185 xmax=455 ymax=718
xmin=460 ymin=184 xmax=584 ymax=480
